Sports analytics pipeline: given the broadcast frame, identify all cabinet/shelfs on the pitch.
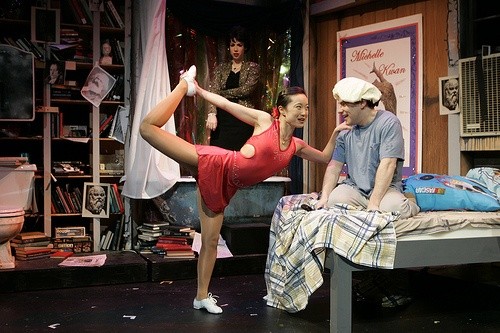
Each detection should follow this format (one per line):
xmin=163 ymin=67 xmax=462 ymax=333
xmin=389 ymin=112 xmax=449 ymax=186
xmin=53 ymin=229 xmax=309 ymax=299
xmin=0 ymin=0 xmax=131 ymax=253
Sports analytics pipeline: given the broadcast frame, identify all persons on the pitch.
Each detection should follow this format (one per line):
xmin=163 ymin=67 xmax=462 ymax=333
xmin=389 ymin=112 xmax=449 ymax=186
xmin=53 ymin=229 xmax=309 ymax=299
xmin=444 ymin=79 xmax=458 ymax=111
xmin=81 ymin=72 xmax=109 ymax=105
xmin=206 ymin=26 xmax=261 ymax=153
xmin=314 ymin=77 xmax=420 ymax=219
xmin=85 ymin=186 xmax=106 ymax=215
xmin=47 ymin=61 xmax=63 ymax=84
xmin=139 ymin=64 xmax=352 ymax=314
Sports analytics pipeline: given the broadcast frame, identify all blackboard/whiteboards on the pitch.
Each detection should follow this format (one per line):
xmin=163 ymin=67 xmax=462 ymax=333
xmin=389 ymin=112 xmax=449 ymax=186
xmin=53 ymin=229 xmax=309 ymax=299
xmin=0 ymin=44 xmax=35 ymax=122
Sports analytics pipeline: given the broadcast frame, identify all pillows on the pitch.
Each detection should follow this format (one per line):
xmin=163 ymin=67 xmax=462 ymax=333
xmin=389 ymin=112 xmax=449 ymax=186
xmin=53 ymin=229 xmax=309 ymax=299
xmin=405 ymin=174 xmax=500 ymax=212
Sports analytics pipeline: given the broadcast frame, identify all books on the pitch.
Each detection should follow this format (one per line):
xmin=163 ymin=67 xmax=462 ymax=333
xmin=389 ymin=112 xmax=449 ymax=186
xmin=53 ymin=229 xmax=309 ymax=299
xmin=0 ymin=0 xmax=124 ymax=139
xmin=0 ymin=150 xmax=196 ymax=269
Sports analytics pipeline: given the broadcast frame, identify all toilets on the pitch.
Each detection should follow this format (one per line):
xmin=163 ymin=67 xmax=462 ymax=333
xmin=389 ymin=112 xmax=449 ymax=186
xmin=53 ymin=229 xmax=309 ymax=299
xmin=0 ymin=207 xmax=25 ymax=270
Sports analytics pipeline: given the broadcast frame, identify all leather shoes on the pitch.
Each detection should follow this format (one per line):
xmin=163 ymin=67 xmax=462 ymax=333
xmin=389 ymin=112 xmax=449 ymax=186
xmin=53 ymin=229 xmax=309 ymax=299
xmin=193 ymin=291 xmax=223 ymax=314
xmin=178 ymin=65 xmax=197 ymax=98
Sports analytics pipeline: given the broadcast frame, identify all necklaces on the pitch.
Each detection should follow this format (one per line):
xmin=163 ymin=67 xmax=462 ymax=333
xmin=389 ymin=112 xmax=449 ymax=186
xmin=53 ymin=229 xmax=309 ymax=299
xmin=233 ymin=66 xmax=240 ymax=70
xmin=282 ymin=130 xmax=290 ymax=145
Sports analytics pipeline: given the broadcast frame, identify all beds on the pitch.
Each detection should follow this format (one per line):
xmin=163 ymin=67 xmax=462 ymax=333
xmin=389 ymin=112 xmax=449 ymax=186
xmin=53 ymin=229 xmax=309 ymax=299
xmin=263 ymin=166 xmax=500 ymax=333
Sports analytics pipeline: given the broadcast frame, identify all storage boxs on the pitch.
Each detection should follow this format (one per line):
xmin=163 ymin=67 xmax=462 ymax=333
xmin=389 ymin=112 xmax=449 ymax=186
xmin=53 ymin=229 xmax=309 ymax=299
xmin=54 ymin=226 xmax=85 ymax=238
